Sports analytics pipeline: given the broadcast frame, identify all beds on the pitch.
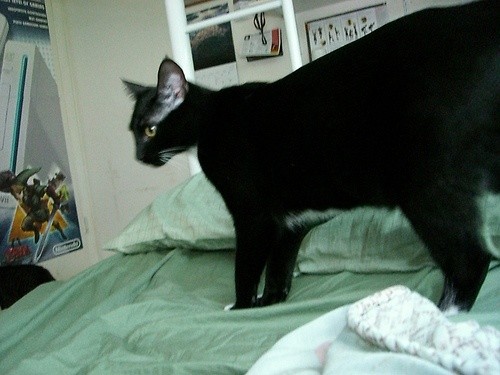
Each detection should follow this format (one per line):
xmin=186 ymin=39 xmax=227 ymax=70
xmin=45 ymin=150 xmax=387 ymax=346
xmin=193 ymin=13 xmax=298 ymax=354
xmin=0 ymin=169 xmax=500 ymax=375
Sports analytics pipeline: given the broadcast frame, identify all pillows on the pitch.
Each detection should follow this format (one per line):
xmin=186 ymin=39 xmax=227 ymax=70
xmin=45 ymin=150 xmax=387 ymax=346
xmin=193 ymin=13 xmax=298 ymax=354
xmin=292 ymin=185 xmax=500 ymax=278
xmin=99 ymin=170 xmax=344 ymax=255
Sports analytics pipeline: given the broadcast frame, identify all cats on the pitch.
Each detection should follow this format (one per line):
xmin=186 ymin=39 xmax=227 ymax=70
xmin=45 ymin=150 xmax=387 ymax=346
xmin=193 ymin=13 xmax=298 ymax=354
xmin=122 ymin=1 xmax=500 ymax=314
